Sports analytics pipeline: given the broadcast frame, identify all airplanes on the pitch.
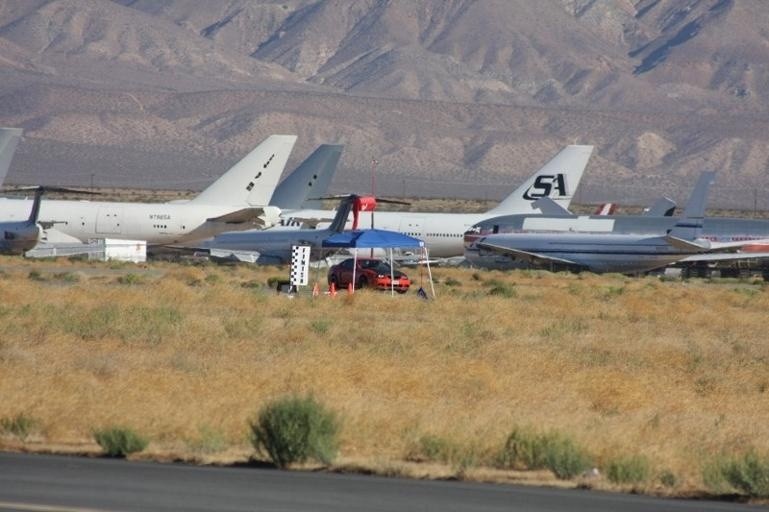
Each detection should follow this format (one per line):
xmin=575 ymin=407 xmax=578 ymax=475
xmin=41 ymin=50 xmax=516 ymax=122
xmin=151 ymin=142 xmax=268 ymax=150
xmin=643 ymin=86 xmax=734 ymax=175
xmin=1 ymin=135 xmax=769 ymax=276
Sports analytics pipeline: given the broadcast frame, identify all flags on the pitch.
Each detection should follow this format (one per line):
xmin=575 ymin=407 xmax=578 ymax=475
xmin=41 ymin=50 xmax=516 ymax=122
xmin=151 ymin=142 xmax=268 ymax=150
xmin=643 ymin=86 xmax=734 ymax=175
xmin=290 ymin=245 xmax=311 ymax=285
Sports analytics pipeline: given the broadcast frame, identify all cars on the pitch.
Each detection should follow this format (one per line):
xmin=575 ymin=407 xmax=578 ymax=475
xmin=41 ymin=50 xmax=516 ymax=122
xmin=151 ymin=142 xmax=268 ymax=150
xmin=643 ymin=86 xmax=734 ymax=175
xmin=328 ymin=256 xmax=411 ymax=295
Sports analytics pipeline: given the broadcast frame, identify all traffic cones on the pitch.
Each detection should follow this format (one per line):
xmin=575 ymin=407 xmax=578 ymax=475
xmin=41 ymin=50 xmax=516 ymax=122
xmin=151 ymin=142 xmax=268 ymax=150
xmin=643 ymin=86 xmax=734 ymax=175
xmin=312 ymin=282 xmax=320 ymax=297
xmin=346 ymin=283 xmax=354 ymax=297
xmin=328 ymin=282 xmax=336 ymax=297
